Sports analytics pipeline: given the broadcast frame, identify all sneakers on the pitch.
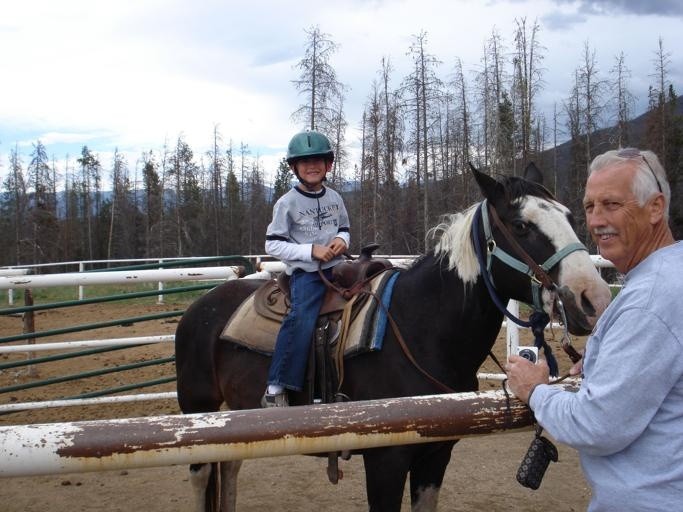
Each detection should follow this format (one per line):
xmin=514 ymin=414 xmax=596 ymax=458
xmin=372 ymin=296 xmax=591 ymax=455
xmin=261 ymin=385 xmax=289 ymax=408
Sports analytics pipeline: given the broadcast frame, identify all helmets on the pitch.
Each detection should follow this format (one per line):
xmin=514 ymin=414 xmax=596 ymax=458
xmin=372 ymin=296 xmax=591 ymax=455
xmin=286 ymin=129 xmax=332 ymax=160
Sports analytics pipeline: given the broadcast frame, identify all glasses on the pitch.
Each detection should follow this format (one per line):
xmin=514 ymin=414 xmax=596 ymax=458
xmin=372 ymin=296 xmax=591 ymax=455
xmin=616 ymin=147 xmax=663 ymax=193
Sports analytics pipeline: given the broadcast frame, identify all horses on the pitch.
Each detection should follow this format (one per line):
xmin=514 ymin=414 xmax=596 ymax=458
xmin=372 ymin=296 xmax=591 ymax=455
xmin=175 ymin=161 xmax=612 ymax=512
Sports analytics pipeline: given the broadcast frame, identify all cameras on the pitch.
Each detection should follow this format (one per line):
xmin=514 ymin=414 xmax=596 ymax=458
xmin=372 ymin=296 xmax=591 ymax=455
xmin=516 ymin=346 xmax=539 ymax=366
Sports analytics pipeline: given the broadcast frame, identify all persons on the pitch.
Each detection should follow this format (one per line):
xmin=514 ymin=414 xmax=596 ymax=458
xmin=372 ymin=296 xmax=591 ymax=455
xmin=503 ymin=144 xmax=683 ymax=512
xmin=256 ymin=131 xmax=352 ymax=408
